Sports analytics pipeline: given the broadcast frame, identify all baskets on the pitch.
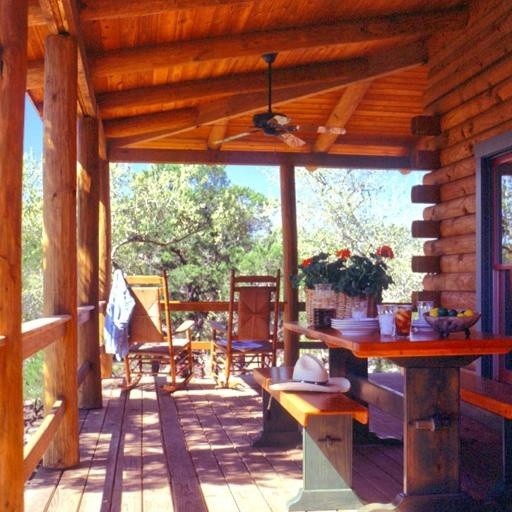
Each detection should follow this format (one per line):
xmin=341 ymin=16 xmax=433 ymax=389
xmin=304 ymin=283 xmax=382 ymax=325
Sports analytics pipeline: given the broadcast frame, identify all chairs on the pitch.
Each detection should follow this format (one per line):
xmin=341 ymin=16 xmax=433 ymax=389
xmin=110 ymin=266 xmax=281 ymax=394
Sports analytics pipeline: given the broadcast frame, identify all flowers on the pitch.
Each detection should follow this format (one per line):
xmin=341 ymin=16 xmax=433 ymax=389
xmin=288 ymin=239 xmax=395 ymax=303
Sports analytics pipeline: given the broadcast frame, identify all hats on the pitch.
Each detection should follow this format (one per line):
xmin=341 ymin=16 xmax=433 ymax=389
xmin=272 ymin=354 xmax=351 ymax=398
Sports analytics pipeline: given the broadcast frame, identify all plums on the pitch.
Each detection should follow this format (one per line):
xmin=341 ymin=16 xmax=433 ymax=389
xmin=437 ymin=308 xmax=447 ymax=316
xmin=448 ymin=308 xmax=457 ymax=317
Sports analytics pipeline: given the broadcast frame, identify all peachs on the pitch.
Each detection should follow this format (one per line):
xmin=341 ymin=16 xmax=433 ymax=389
xmin=464 ymin=307 xmax=474 ymax=317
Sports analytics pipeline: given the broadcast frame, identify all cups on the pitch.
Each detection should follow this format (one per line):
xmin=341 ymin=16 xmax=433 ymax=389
xmin=352 ymin=300 xmax=368 ymax=318
xmin=377 ymin=304 xmax=412 ymax=337
xmin=272 ymin=366 xmax=288 ymax=382
xmin=416 ymin=300 xmax=434 ymax=320
xmin=314 ymin=282 xmax=332 ymax=308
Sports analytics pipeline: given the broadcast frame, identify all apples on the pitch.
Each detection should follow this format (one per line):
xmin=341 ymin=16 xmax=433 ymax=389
xmin=429 ymin=307 xmax=439 ymax=317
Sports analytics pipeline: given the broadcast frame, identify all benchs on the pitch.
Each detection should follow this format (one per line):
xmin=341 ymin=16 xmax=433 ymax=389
xmin=249 ymin=366 xmax=369 ymax=511
xmin=459 ymin=367 xmax=512 ymax=483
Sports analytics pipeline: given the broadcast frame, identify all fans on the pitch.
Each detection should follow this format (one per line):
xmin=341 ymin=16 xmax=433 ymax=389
xmin=198 ymin=53 xmax=347 ymax=147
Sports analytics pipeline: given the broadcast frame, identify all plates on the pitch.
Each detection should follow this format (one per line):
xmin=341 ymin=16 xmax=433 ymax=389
xmin=314 ymin=309 xmax=336 ymax=328
xmin=330 ymin=317 xmax=378 ymax=337
